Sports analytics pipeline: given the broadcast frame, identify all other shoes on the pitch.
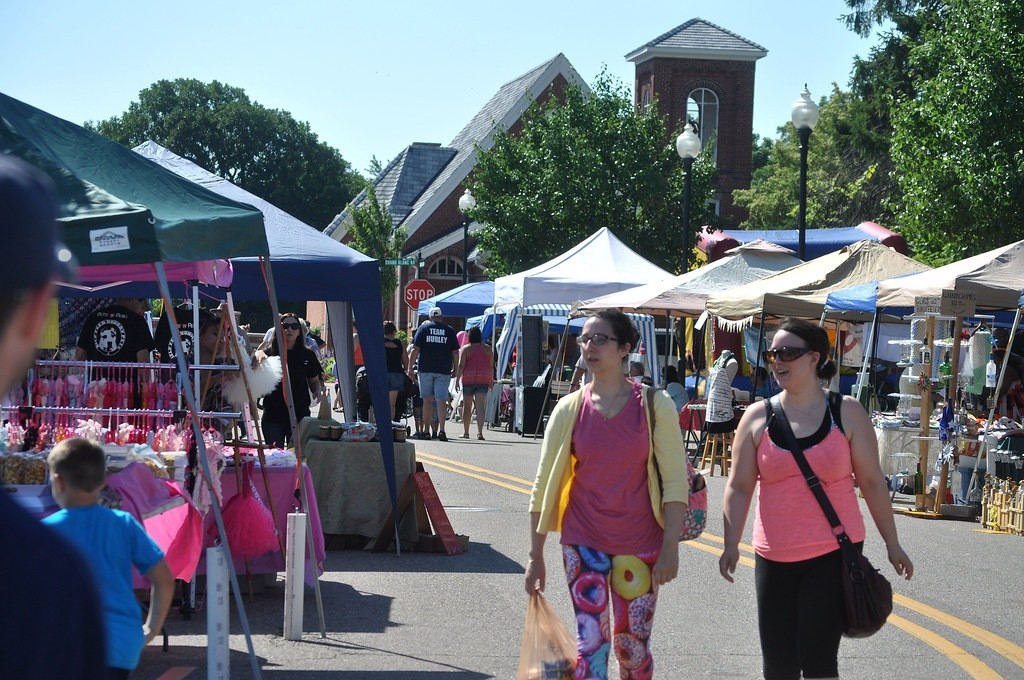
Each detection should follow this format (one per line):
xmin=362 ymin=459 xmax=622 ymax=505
xmin=458 ymin=434 xmax=469 ymax=438
xmin=477 ymin=434 xmax=485 ymax=440
xmin=413 ymin=431 xmax=438 ymax=439
xmin=333 ymin=406 xmax=344 ymax=412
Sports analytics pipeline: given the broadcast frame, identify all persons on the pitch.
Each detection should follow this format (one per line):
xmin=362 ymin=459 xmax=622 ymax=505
xmin=41 ymin=438 xmax=173 ymax=680
xmin=406 ymin=307 xmax=494 ymax=440
xmin=0 ymin=156 xmax=109 ymax=680
xmin=182 ymin=316 xmax=259 ymax=438
xmin=751 ymin=367 xmax=771 ymax=398
xmin=257 ymin=312 xmax=327 ymax=450
xmin=524 ymin=309 xmax=689 ymax=680
xmin=569 ymin=355 xmax=629 ymax=393
xmin=333 ymin=320 xmax=410 ymax=421
xmin=971 ymin=329 xmax=1024 ymax=414
xmin=720 ymin=317 xmax=913 ymax=680
xmin=544 ymin=338 xmax=568 ymax=381
xmin=630 ymin=361 xmax=688 ymax=412
xmin=702 ymin=350 xmax=738 ymax=434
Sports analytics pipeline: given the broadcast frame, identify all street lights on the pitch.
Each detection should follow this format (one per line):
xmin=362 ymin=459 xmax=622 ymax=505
xmin=458 ymin=187 xmax=476 ymax=282
xmin=790 ymin=84 xmax=821 ymax=261
xmin=676 ymin=124 xmax=701 ymax=273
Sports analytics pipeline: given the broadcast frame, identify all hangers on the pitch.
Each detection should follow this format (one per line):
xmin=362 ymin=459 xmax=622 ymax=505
xmin=0 ymin=357 xmax=218 ymax=442
xmin=969 ymin=317 xmax=992 ymax=337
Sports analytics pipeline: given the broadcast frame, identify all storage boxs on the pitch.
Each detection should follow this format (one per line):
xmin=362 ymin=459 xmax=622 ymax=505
xmin=958 ymin=455 xmax=986 ymax=470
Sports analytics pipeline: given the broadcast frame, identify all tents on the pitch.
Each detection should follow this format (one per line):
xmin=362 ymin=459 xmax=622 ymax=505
xmin=415 ymin=228 xmax=679 ymax=363
xmin=0 ymin=92 xmax=325 ymax=680
xmin=703 ymin=240 xmax=1024 ymax=503
xmin=57 ymin=139 xmax=401 ymax=557
xmin=534 ymin=238 xmax=805 ymax=438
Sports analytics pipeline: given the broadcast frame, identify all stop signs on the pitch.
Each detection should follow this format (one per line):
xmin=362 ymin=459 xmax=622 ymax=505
xmin=404 ymin=279 xmax=436 ymax=311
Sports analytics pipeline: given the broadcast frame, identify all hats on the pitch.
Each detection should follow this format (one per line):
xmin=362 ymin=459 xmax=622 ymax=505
xmin=429 ymin=307 xmax=442 ymax=317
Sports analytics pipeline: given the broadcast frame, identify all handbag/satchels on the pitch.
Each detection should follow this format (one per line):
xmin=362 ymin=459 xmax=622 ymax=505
xmin=645 ymin=387 xmax=710 ymax=539
xmin=836 ymin=530 xmax=893 ymax=638
xmin=516 ymin=591 xmax=577 ymax=680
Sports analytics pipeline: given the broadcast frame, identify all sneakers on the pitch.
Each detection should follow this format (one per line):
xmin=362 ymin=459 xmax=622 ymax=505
xmin=418 ymin=433 xmax=431 ymax=440
xmin=438 ymin=431 xmax=449 ymax=441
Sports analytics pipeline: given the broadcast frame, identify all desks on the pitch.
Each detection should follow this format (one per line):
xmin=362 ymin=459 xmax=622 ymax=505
xmin=683 ymin=403 xmax=747 ymax=466
xmin=160 ymin=447 xmax=325 ymax=601
xmin=299 ymin=437 xmax=419 ymax=548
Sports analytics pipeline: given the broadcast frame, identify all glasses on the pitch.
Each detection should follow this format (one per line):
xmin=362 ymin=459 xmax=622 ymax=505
xmin=761 ymin=346 xmax=812 ymax=364
xmin=575 ymin=332 xmax=621 ymax=345
xmin=281 ymin=322 xmax=302 ymax=330
